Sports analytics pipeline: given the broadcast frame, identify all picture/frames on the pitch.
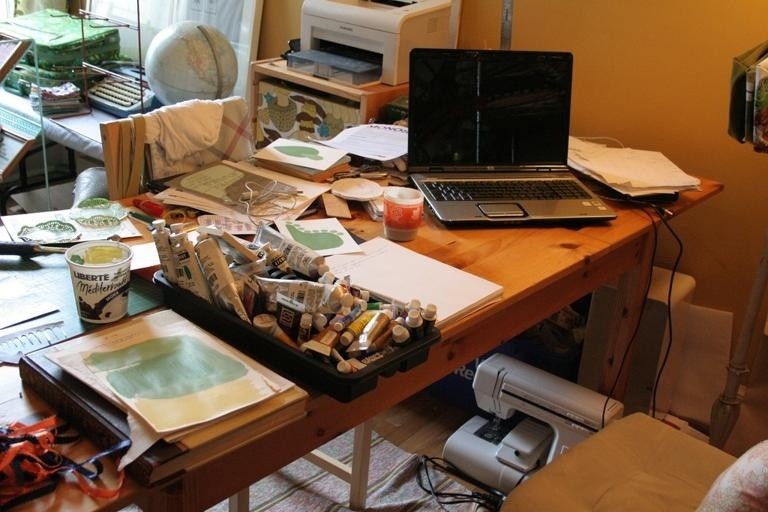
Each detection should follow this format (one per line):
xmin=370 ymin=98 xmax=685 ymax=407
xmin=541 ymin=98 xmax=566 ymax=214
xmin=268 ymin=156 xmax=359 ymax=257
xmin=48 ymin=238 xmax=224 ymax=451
xmin=0 ymin=69 xmax=138 ymax=215
xmin=0 ymin=140 xmax=726 ymax=512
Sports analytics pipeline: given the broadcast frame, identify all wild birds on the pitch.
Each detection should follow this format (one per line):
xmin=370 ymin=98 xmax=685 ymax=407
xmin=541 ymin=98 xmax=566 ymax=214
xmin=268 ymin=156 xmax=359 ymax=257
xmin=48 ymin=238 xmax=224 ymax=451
xmin=406 ymin=47 xmax=617 ymax=224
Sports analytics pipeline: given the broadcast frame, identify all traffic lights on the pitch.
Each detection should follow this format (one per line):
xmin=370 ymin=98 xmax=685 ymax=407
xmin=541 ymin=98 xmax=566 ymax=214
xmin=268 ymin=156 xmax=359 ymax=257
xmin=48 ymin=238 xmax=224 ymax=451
xmin=690 ymin=436 xmax=768 ymax=512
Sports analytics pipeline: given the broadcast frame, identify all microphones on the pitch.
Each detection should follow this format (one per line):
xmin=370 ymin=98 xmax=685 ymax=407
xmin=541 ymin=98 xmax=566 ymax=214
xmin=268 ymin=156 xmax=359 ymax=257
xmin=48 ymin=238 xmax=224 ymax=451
xmin=486 ymin=405 xmax=743 ymax=512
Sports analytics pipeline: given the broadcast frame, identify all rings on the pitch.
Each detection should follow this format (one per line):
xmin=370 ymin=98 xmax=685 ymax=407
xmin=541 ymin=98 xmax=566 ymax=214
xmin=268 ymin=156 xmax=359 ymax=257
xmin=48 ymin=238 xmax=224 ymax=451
xmin=129 ymin=211 xmax=171 ymax=228
xmin=241 ymin=191 xmax=303 ymax=200
xmin=337 ymin=172 xmax=388 ymax=179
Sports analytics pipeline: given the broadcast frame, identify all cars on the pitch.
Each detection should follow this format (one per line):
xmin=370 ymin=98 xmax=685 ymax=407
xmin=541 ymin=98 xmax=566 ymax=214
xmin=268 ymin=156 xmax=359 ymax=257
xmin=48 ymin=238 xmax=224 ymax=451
xmin=383 ymin=187 xmax=424 ymax=242
xmin=63 ymin=240 xmax=133 ymax=324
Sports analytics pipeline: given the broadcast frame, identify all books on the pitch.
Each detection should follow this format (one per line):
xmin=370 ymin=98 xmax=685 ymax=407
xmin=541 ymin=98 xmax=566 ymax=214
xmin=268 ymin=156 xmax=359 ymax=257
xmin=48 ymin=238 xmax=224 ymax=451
xmin=322 ymin=235 xmax=504 ymax=330
xmin=18 ymin=304 xmax=309 ymax=489
xmin=155 ymin=139 xmax=351 ymax=230
xmin=27 ymin=81 xmax=81 ymax=115
xmin=358 ymin=173 xmax=425 ymax=223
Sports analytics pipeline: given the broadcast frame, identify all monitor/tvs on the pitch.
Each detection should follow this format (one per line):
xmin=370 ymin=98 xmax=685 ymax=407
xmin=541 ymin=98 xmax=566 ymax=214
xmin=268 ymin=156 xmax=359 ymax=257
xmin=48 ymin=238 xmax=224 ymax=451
xmin=27 ymin=81 xmax=81 ymax=115
xmin=322 ymin=235 xmax=504 ymax=330
xmin=358 ymin=173 xmax=425 ymax=223
xmin=155 ymin=139 xmax=351 ymax=230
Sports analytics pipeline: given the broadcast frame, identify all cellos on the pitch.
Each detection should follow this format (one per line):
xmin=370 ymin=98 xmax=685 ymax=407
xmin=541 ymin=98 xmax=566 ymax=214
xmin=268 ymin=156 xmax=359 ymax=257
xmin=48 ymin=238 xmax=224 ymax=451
xmin=287 ymin=0 xmax=452 ymax=89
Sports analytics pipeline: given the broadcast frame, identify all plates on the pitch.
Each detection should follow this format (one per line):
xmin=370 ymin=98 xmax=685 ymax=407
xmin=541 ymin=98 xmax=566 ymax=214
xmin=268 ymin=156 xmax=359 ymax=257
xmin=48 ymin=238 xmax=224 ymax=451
xmin=237 ymin=51 xmax=411 ymax=147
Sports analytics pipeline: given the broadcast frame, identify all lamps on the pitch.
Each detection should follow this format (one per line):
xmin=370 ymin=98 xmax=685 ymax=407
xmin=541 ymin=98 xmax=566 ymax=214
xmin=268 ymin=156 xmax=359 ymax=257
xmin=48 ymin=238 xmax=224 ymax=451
xmin=96 ymin=94 xmax=258 ymax=204
